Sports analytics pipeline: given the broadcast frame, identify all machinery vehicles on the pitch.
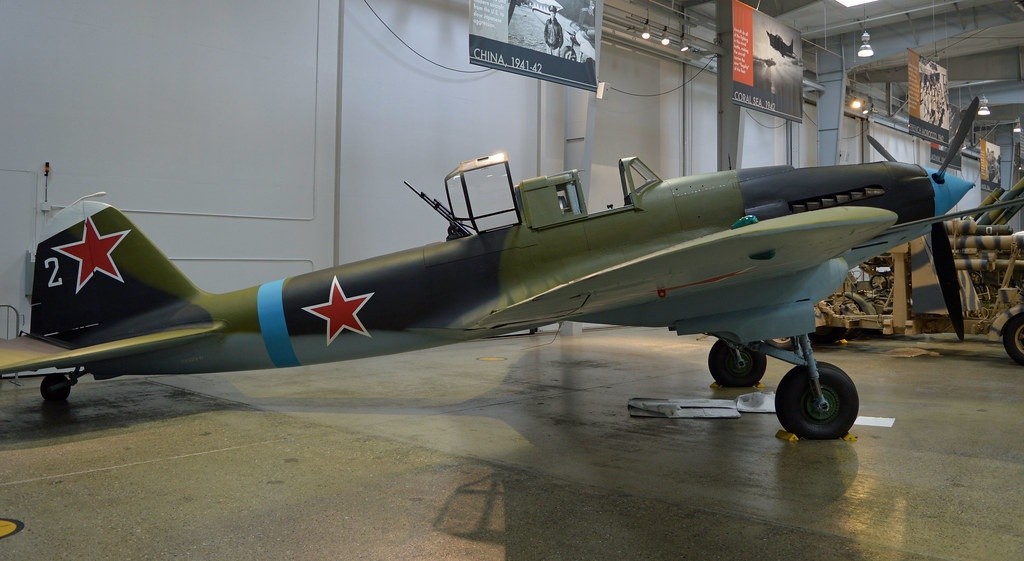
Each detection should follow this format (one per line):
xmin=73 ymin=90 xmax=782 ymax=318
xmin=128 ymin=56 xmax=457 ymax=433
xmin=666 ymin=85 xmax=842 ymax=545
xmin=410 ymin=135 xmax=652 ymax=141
xmin=766 ymin=175 xmax=1024 ymax=369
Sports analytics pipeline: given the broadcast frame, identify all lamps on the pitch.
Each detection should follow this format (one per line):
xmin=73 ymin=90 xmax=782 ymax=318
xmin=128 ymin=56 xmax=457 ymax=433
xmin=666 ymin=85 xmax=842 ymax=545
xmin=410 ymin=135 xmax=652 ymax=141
xmin=962 ymin=144 xmax=966 ymax=149
xmin=642 ymin=18 xmax=650 ymax=40
xmin=858 ymin=5 xmax=874 ymax=57
xmin=978 ymin=94 xmax=990 ymax=115
xmin=661 ymin=25 xmax=670 ymax=46
xmin=681 ymin=25 xmax=689 ymax=52
xmin=1013 ymin=118 xmax=1021 ymax=132
xmin=862 ymin=107 xmax=868 ymax=115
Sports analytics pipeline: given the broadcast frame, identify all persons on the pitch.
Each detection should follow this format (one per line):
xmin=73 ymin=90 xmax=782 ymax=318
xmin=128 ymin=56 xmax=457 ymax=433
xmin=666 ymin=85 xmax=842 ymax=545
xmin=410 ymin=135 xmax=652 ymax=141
xmin=986 ymin=148 xmax=1000 ymax=182
xmin=543 ymin=5 xmax=564 ymax=56
xmin=920 ymin=79 xmax=948 ymax=127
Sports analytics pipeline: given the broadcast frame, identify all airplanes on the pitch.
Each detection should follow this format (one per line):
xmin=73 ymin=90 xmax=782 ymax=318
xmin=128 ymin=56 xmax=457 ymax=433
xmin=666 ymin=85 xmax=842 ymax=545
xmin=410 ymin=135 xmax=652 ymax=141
xmin=506 ymin=0 xmax=595 ymax=65
xmin=0 ymin=93 xmax=1024 ymax=442
xmin=766 ymin=29 xmax=799 ymax=60
